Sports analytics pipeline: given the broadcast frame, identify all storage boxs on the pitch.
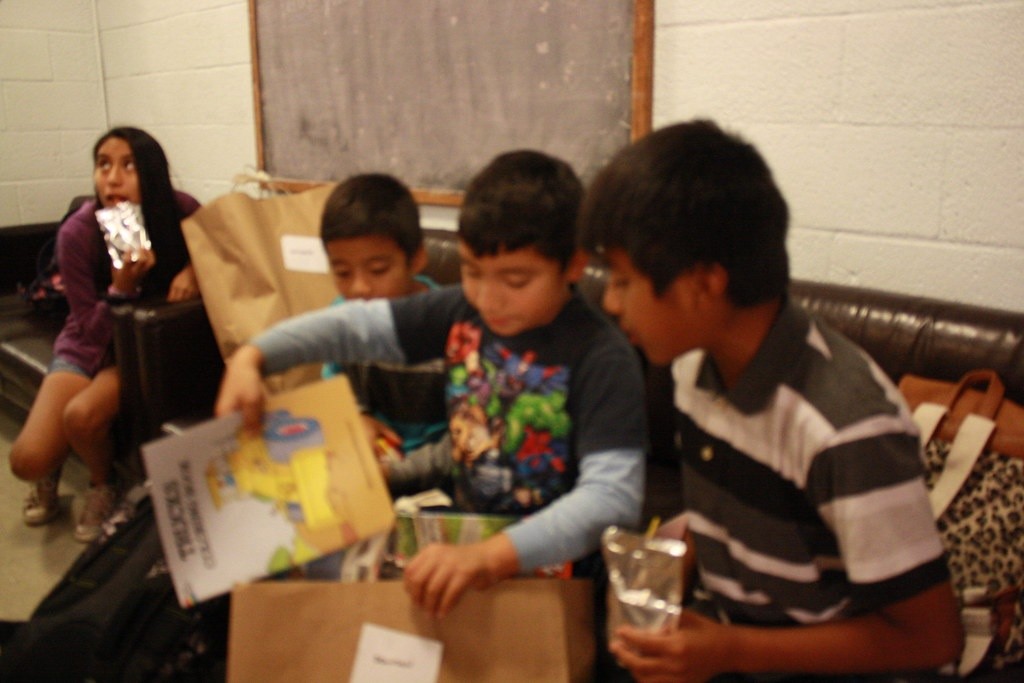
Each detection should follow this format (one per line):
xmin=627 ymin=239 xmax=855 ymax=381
xmin=228 ymin=571 xmax=592 ymax=683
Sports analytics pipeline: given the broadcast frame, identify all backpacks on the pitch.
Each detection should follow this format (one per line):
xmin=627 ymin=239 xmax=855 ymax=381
xmin=0 ymin=494 xmax=227 ymax=683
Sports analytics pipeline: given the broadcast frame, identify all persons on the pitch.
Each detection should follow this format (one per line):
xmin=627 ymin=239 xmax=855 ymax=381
xmin=319 ymin=174 xmax=457 ymax=516
xmin=576 ymin=120 xmax=964 ymax=683
xmin=8 ymin=128 xmax=204 ymax=541
xmin=216 ymin=149 xmax=650 ymax=621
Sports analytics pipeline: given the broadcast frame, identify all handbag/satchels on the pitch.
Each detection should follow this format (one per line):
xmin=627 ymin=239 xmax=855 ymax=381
xmin=898 ymin=370 xmax=1024 ymax=460
xmin=912 ymin=401 xmax=1024 ymax=678
xmin=182 ymin=174 xmax=341 ymax=394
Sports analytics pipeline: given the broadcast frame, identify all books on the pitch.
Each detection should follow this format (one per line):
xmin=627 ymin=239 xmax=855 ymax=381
xmin=142 ymin=373 xmax=397 ymax=607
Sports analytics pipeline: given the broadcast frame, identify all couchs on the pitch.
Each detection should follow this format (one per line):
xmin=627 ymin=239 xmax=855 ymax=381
xmin=0 ymin=190 xmax=1024 ymax=683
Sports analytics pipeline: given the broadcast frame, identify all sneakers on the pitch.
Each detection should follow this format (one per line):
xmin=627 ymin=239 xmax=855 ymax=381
xmin=23 ymin=492 xmax=60 ymax=524
xmin=75 ymin=476 xmax=117 ymax=540
